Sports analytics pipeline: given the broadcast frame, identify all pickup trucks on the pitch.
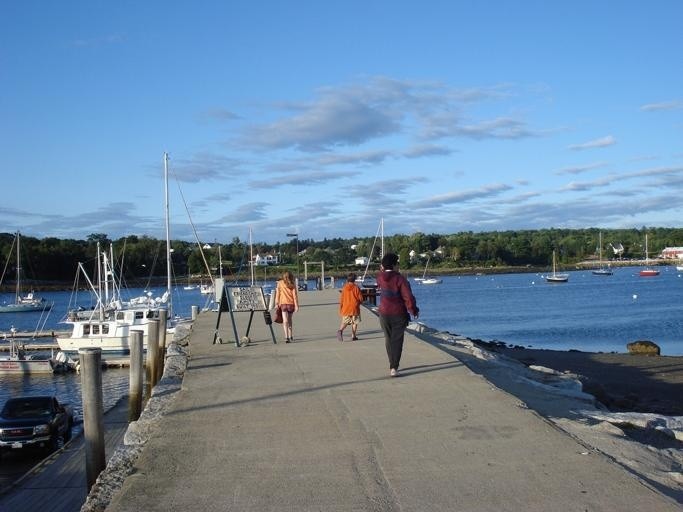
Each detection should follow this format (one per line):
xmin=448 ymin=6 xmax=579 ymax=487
xmin=0 ymin=395 xmax=73 ymax=455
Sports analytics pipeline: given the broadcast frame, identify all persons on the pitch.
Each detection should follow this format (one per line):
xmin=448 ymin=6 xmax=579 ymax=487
xmin=275 ymin=271 xmax=299 ymax=344
xmin=336 ymin=272 xmax=364 ymax=342
xmin=377 ymin=253 xmax=419 ymax=377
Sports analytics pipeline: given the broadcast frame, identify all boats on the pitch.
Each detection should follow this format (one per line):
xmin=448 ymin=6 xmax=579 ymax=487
xmin=546 ymin=251 xmax=568 ymax=282
xmin=592 ymin=232 xmax=614 ymax=275
xmin=414 ymin=258 xmax=443 ymax=284
xmin=640 ymin=234 xmax=660 ymax=276
xmin=355 ymin=218 xmax=383 ymax=296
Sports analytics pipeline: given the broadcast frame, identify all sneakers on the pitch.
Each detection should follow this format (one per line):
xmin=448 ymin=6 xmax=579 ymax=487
xmin=390 ymin=368 xmax=401 ymax=377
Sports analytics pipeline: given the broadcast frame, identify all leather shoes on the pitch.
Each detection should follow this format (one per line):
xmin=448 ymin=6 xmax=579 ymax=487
xmin=285 ymin=336 xmax=293 ymax=344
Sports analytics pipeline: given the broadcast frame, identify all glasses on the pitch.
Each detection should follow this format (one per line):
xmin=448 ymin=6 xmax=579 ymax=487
xmin=397 ymin=261 xmax=399 ymax=264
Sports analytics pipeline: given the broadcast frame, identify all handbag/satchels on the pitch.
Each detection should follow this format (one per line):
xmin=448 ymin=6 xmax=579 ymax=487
xmin=406 ymin=313 xmax=411 ymax=326
xmin=273 ymin=307 xmax=283 ymax=323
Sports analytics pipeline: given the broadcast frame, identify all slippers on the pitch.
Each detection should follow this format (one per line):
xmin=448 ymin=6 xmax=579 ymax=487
xmin=352 ymin=336 xmax=358 ymax=341
xmin=337 ymin=330 xmax=343 ymax=344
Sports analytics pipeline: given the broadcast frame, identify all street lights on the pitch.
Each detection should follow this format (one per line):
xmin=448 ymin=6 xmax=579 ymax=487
xmin=287 ymin=234 xmax=299 ymax=282
